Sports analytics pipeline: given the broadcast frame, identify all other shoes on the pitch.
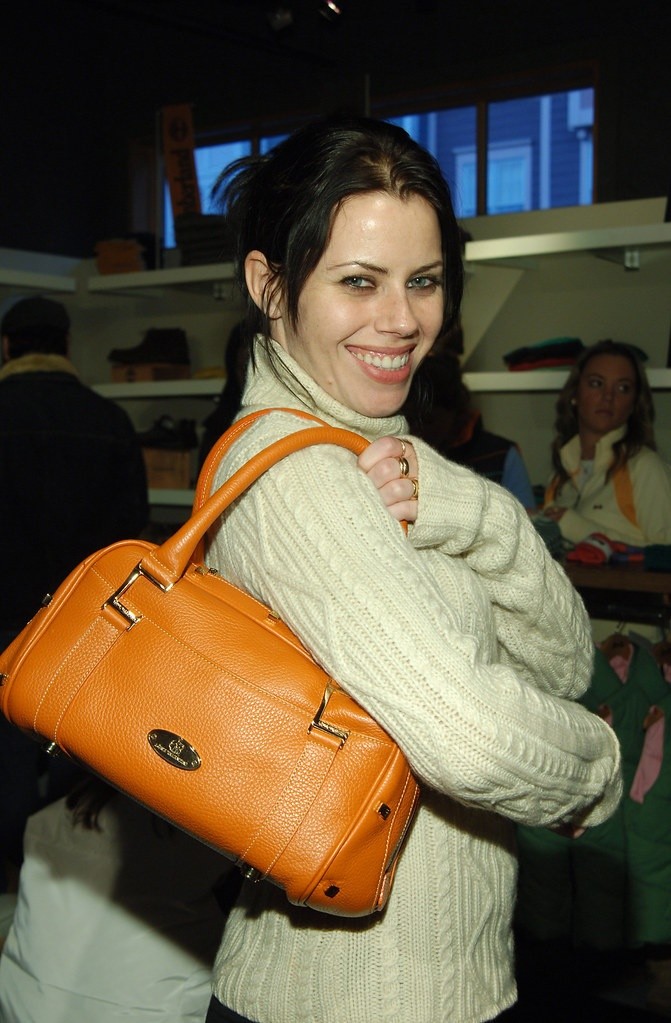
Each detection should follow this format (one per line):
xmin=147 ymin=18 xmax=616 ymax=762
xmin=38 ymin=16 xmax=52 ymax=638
xmin=107 ymin=326 xmax=189 ymax=368
xmin=135 ymin=416 xmax=198 ymax=450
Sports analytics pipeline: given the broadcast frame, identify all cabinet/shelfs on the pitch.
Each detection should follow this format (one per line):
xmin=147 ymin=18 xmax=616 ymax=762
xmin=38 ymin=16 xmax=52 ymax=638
xmin=0 ymin=196 xmax=671 ymax=507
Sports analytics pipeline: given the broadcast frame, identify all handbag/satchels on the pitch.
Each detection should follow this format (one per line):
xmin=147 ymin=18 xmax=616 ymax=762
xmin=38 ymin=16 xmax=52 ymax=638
xmin=0 ymin=407 xmax=422 ymax=919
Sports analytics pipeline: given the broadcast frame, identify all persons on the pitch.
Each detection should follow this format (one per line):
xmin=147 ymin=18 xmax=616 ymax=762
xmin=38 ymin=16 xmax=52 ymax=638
xmin=441 ymin=409 xmax=537 ymax=512
xmin=0 ymin=295 xmax=149 ymax=895
xmin=540 ymin=339 xmax=671 ymax=547
xmin=192 ymin=313 xmax=273 ymax=492
xmin=200 ymin=120 xmax=625 ymax=1023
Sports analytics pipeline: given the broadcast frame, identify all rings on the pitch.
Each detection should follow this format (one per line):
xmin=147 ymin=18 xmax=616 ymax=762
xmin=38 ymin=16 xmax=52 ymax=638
xmin=395 ymin=457 xmax=409 ymax=479
xmin=411 ymin=479 xmax=418 ymax=498
xmin=396 ymin=437 xmax=406 ymax=457
